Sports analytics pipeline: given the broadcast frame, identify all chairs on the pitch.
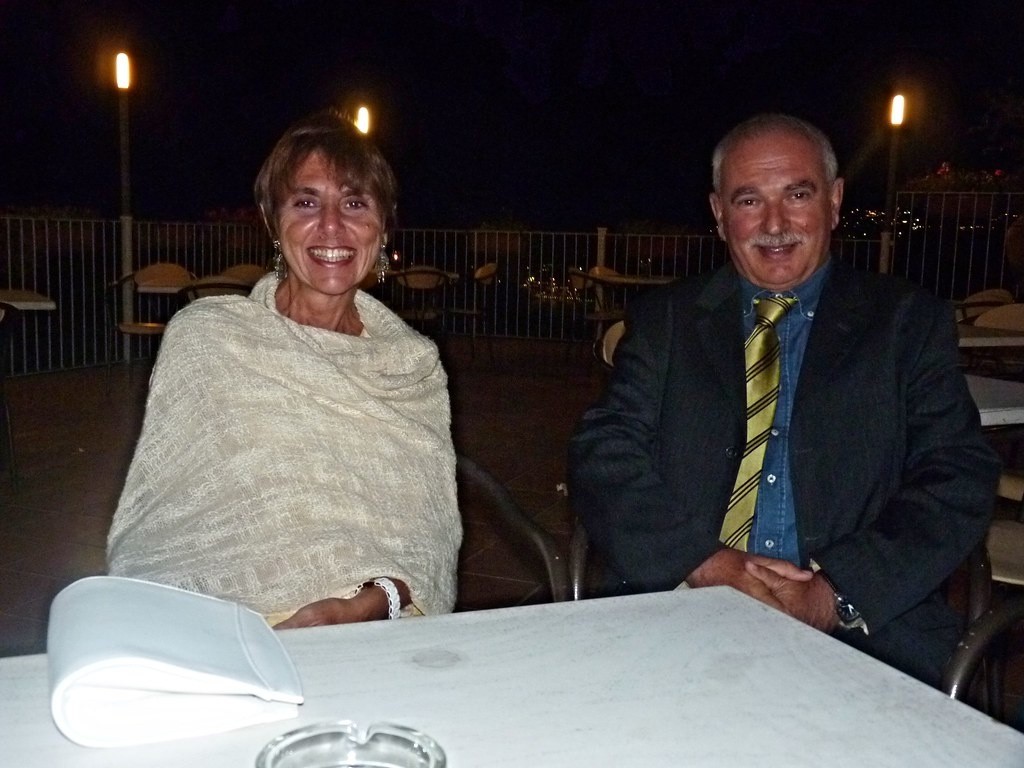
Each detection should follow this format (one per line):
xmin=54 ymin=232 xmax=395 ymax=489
xmin=388 ymin=266 xmax=460 ymax=359
xmin=566 ymin=267 xmax=628 ymax=357
xmin=954 ymin=289 xmax=1013 ymax=323
xmin=450 ymin=458 xmax=555 ymax=615
xmin=972 ymin=304 xmax=1024 ymax=382
xmin=940 ymin=595 xmax=1024 ymax=734
xmin=571 ymin=523 xmax=1002 ymax=712
xmin=179 ymin=275 xmax=255 ymax=306
xmin=108 ymin=264 xmax=196 ymax=391
xmin=222 ymin=263 xmax=270 ymax=286
xmin=434 ymin=264 xmax=500 ymax=363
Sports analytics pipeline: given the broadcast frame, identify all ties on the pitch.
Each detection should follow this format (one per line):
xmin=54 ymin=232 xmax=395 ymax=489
xmin=717 ymin=295 xmax=799 ymax=552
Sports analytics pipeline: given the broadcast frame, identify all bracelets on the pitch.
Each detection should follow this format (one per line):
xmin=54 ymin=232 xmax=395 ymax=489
xmin=354 ymin=576 xmax=404 ymax=621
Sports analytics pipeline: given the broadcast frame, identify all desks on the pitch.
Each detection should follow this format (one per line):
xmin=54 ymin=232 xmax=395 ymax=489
xmin=0 ymin=585 xmax=1024 ymax=768
xmin=965 ymin=373 xmax=1024 ymax=428
xmin=957 ymin=323 xmax=1024 ymax=378
xmin=0 ymin=289 xmax=56 ymax=496
xmin=598 ymin=273 xmax=676 ymax=365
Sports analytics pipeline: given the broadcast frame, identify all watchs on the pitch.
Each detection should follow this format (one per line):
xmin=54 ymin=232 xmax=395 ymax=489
xmin=816 ymin=568 xmax=862 ymax=624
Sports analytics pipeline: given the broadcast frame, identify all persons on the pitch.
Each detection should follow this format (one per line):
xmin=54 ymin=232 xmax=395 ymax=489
xmin=561 ymin=113 xmax=999 ymax=693
xmin=109 ymin=111 xmax=465 ymax=631
